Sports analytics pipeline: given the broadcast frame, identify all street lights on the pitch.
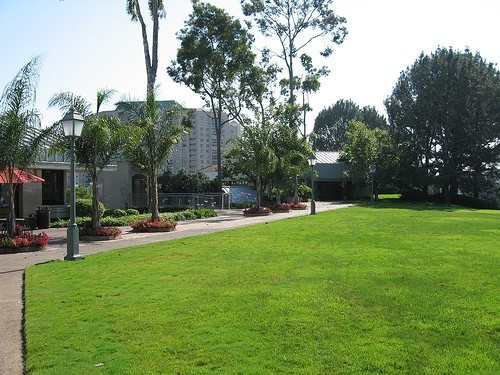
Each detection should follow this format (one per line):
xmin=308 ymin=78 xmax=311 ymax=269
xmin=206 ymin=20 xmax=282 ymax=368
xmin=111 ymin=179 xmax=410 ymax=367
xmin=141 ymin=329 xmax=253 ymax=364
xmin=306 ymin=154 xmax=316 ymax=215
xmin=369 ymin=163 xmax=376 ymax=203
xmin=59 ymin=104 xmax=84 ymax=262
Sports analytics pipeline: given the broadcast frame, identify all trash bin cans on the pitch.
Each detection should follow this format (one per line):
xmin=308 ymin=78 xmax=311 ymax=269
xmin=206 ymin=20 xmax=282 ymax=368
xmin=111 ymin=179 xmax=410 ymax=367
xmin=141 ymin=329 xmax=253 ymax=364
xmin=35 ymin=206 xmax=52 ymax=229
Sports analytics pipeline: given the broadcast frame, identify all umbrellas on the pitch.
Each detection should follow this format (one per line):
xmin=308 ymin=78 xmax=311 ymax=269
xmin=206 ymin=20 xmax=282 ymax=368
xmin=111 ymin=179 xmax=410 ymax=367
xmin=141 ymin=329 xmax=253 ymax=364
xmin=0 ymin=161 xmax=46 ymax=183
